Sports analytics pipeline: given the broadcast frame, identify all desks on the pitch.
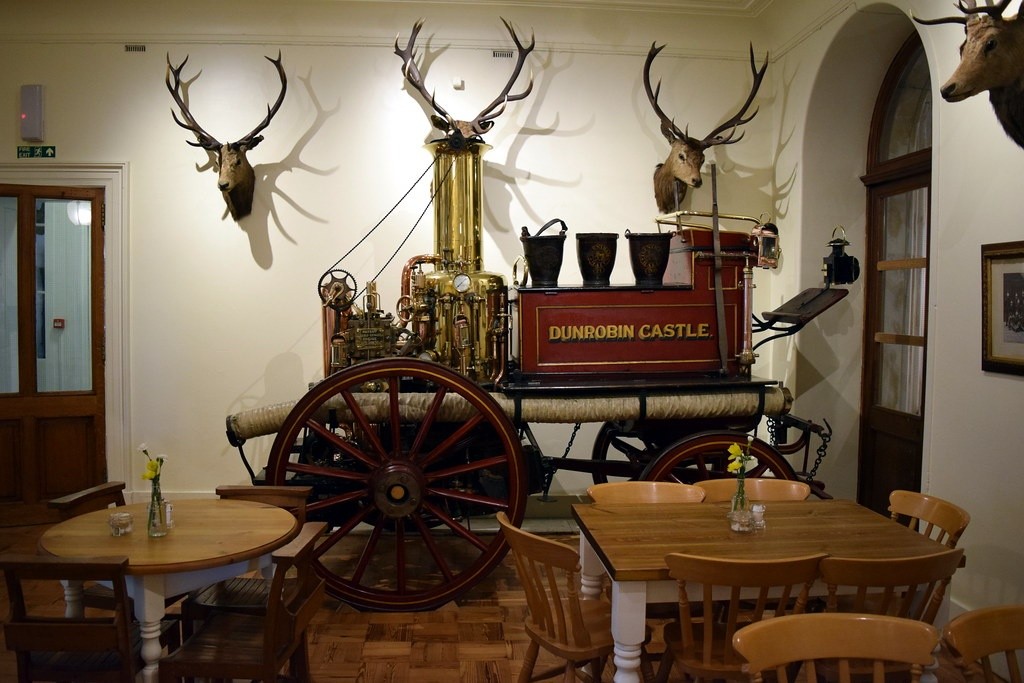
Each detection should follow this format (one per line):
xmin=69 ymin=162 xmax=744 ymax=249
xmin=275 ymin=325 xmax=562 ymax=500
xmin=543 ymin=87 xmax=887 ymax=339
xmin=571 ymin=498 xmax=966 ymax=683
xmin=41 ymin=499 xmax=300 ymax=683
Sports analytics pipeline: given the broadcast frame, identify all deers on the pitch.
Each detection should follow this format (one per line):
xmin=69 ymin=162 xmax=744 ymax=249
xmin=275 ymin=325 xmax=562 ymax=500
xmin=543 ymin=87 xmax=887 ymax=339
xmin=166 ymin=49 xmax=287 ymax=222
xmin=643 ymin=40 xmax=769 ymax=215
xmin=394 ymin=16 xmax=535 ymax=152
xmin=910 ymin=0 xmax=1024 ymax=149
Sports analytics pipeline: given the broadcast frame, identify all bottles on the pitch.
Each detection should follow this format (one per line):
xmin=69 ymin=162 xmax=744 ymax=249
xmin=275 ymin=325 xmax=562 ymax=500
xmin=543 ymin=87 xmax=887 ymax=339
xmin=109 ymin=512 xmax=133 ymax=535
xmin=147 ymin=500 xmax=173 ymax=529
xmin=751 ymin=504 xmax=768 ymax=529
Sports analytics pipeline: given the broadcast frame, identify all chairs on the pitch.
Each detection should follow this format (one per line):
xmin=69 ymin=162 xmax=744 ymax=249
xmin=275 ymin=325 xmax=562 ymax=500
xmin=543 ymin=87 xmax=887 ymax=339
xmin=0 ymin=478 xmax=1024 ymax=683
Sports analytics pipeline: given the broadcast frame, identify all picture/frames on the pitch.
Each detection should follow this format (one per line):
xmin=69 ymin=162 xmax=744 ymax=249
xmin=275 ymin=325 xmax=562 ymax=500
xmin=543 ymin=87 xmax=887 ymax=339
xmin=981 ymin=240 xmax=1024 ymax=378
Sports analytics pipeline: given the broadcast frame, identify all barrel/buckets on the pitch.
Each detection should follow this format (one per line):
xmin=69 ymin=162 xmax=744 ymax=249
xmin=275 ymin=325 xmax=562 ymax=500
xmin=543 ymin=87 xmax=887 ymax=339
xmin=622 ymin=229 xmax=676 ymax=284
xmin=520 ymin=218 xmax=567 ymax=288
xmin=576 ymin=231 xmax=618 ymax=286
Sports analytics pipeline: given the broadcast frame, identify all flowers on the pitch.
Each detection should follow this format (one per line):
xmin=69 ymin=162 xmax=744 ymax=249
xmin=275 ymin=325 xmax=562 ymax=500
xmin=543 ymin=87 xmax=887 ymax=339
xmin=726 ymin=435 xmax=759 ymax=513
xmin=137 ymin=442 xmax=168 ymax=529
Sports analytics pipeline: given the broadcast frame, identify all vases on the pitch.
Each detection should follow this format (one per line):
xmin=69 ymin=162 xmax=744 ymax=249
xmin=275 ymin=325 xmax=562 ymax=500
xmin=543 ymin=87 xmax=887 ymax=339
xmin=575 ymin=233 xmax=619 ymax=287
xmin=146 ymin=484 xmax=167 ymax=537
xmin=728 ymin=480 xmax=752 ymax=531
xmin=624 ymin=228 xmax=675 ymax=285
xmin=519 ymin=226 xmax=567 ymax=288
